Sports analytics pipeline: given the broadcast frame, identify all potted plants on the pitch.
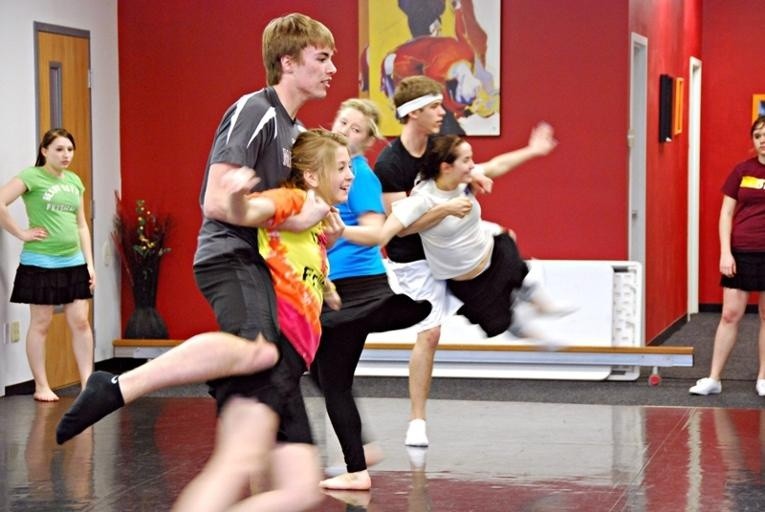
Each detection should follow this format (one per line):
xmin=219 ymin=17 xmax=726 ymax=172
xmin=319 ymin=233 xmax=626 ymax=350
xmin=112 ymin=192 xmax=172 ymax=339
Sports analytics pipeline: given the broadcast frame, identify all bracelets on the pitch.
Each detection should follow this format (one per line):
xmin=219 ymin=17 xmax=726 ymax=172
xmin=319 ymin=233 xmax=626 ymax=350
xmin=321 ymin=283 xmax=336 ymax=296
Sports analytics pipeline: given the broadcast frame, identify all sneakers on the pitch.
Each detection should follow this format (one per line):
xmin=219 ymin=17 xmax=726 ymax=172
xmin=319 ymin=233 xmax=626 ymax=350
xmin=688 ymin=377 xmax=722 ymax=395
xmin=755 ymin=379 xmax=764 ymax=397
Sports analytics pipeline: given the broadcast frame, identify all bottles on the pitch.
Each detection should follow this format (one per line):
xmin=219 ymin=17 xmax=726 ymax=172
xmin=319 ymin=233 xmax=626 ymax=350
xmin=648 ymin=366 xmax=661 ymax=386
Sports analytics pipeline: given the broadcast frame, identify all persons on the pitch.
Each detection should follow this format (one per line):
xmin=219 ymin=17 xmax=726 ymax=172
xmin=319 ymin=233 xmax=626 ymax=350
xmin=0 ymin=128 xmax=96 ymax=402
xmin=57 ymin=13 xmax=345 ymax=511
xmin=688 ymin=115 xmax=764 ymax=396
xmin=373 ymin=73 xmax=495 ymax=448
xmin=309 ymin=100 xmax=432 ymax=489
xmin=377 ymin=122 xmax=575 ymax=340
xmin=171 ymin=126 xmax=355 ymax=512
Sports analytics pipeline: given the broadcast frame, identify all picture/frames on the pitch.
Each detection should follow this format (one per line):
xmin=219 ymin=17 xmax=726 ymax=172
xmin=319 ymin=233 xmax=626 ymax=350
xmin=659 ymin=74 xmax=672 ymax=142
xmin=672 ymin=78 xmax=684 ymax=135
xmin=752 ymin=94 xmax=765 ymax=128
